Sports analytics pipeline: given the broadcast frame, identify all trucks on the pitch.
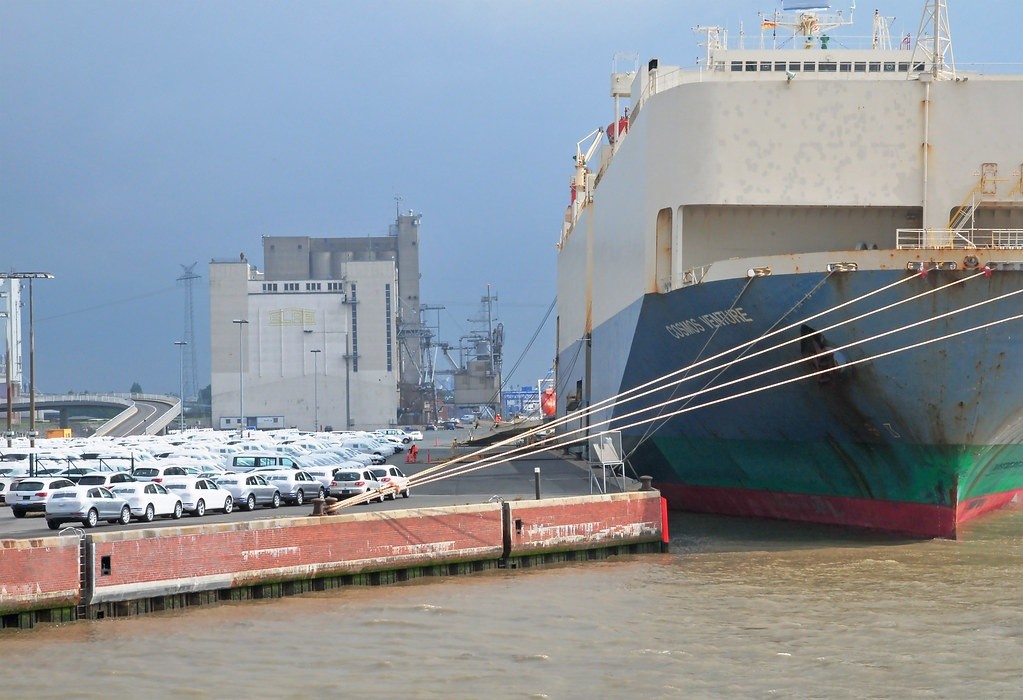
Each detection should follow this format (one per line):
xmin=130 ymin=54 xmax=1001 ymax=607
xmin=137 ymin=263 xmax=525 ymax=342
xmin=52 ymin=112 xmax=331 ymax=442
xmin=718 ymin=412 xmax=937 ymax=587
xmin=461 ymin=415 xmax=474 ymax=421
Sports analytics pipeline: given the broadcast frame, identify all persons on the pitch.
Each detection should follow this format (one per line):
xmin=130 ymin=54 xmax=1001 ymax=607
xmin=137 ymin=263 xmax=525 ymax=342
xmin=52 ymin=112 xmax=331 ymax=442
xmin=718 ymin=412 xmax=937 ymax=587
xmin=451 ymin=439 xmax=457 ymax=455
xmin=496 ymin=414 xmax=501 ymax=427
xmin=410 ymin=445 xmax=418 ymax=463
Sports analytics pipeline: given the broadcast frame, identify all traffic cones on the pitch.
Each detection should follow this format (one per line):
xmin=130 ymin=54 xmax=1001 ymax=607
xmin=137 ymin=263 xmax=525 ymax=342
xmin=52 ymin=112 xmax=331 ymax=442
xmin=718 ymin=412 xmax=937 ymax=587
xmin=428 ymin=454 xmax=430 ymax=464
xmin=436 ymin=437 xmax=439 ymax=447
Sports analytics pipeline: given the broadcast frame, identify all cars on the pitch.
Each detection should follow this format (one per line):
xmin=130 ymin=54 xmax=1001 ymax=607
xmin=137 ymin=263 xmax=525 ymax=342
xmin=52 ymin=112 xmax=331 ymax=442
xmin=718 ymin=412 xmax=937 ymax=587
xmin=330 ymin=469 xmax=384 ymax=505
xmin=426 ymin=424 xmax=434 ymax=431
xmin=0 ymin=425 xmax=412 ymax=528
xmin=444 ymin=422 xmax=454 ymax=431
xmin=411 ymin=431 xmax=423 ymax=441
xmin=366 ymin=465 xmax=412 ymax=500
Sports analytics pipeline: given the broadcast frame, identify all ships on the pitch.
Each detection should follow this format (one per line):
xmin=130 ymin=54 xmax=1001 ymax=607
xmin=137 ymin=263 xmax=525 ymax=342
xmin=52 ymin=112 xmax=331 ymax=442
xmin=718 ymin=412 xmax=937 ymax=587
xmin=552 ymin=1 xmax=1023 ymax=540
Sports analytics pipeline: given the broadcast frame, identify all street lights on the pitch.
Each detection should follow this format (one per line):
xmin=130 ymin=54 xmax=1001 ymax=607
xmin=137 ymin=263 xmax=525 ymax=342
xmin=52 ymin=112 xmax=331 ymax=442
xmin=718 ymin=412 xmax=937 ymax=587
xmin=174 ymin=341 xmax=188 ymax=434
xmin=9 ymin=272 xmax=55 ymax=447
xmin=310 ymin=350 xmax=322 ymax=432
xmin=234 ymin=319 xmax=249 ymax=438
